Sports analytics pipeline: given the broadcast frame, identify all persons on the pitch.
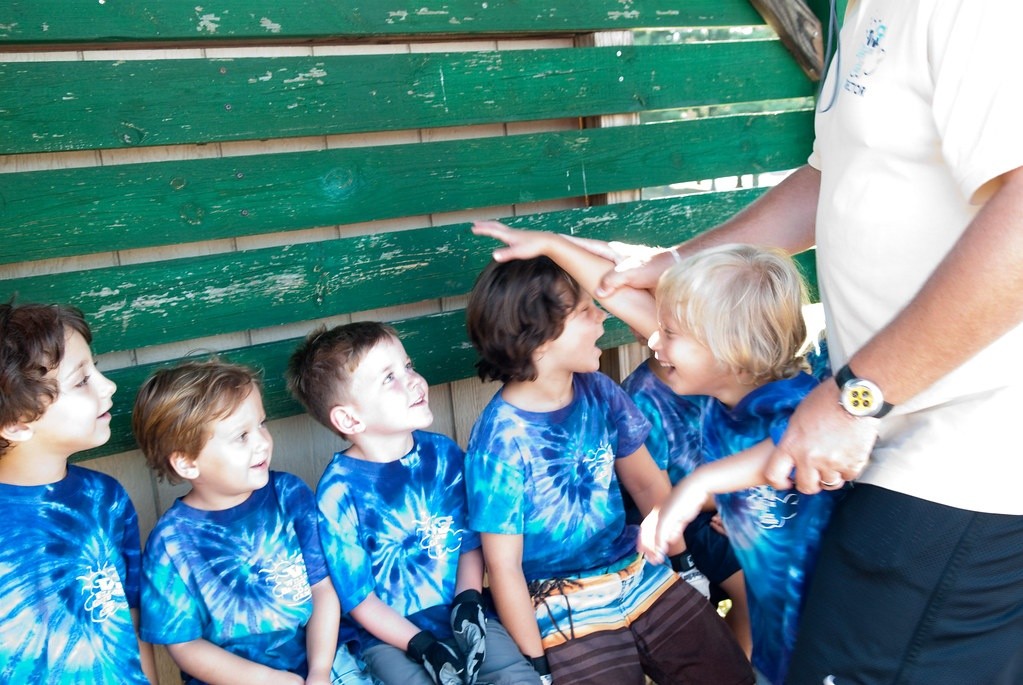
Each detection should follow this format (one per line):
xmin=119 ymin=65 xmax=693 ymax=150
xmin=463 ymin=254 xmax=759 ymax=684
xmin=472 ymin=218 xmax=837 ymax=684
xmin=286 ymin=320 xmax=542 ymax=685
xmin=133 ymin=362 xmax=382 ymax=685
xmin=558 ymin=0 xmax=1023 ymax=685
xmin=0 ymin=304 xmax=151 ymax=685
xmin=620 ymin=334 xmax=753 ymax=662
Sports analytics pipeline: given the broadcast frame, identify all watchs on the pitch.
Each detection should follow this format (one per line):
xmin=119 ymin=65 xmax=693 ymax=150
xmin=835 ymin=364 xmax=893 ymax=419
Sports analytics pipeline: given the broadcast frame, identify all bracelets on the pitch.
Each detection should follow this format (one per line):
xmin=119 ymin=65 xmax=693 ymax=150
xmin=668 ymin=248 xmax=681 ymax=263
